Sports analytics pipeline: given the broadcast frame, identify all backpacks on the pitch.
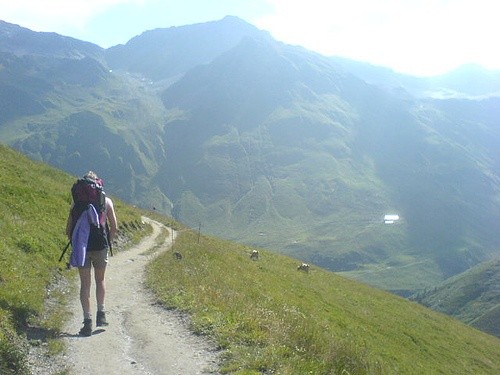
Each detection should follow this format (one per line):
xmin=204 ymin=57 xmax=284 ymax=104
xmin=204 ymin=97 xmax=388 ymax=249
xmin=70 ymin=176 xmax=109 ymax=250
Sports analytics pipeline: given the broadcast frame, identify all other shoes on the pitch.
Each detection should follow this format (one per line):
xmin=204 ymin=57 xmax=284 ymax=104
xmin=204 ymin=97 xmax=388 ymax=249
xmin=96 ymin=311 xmax=109 ymax=326
xmin=80 ymin=318 xmax=92 ymax=336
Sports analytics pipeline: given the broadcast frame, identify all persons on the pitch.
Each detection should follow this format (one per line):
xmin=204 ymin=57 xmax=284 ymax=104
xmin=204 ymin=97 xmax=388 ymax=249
xmin=66 ymin=171 xmax=118 ymax=335
